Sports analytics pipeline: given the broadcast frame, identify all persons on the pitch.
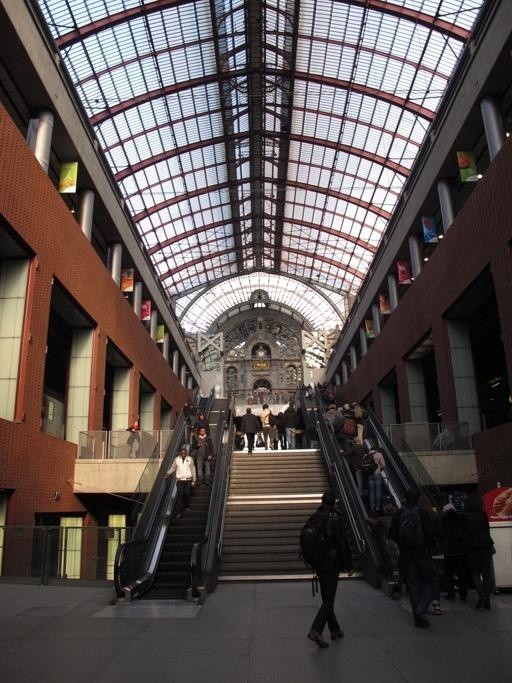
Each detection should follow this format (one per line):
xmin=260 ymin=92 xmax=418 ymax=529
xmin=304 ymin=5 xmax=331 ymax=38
xmin=307 ymin=488 xmax=496 ymax=647
xmin=302 ymin=382 xmax=387 ymax=517
xmin=239 ymin=391 xmax=306 ymax=454
xmin=193 ymin=429 xmax=214 ymax=486
xmin=126 ymin=420 xmax=140 ymax=458
xmin=193 ymin=414 xmax=211 ymax=437
xmin=164 ymin=448 xmax=197 ymax=519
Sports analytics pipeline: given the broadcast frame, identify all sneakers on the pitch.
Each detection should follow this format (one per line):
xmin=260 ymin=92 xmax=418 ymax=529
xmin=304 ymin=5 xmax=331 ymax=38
xmin=331 ymin=631 xmax=345 ymax=640
xmin=308 ymin=631 xmax=330 ymax=648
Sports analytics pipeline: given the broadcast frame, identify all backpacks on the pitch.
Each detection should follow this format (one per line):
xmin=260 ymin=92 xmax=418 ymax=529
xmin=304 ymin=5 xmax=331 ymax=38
xmin=396 ymin=505 xmax=425 ymax=548
xmin=298 ymin=511 xmax=332 ymax=574
xmin=360 ymin=452 xmax=377 ymax=476
